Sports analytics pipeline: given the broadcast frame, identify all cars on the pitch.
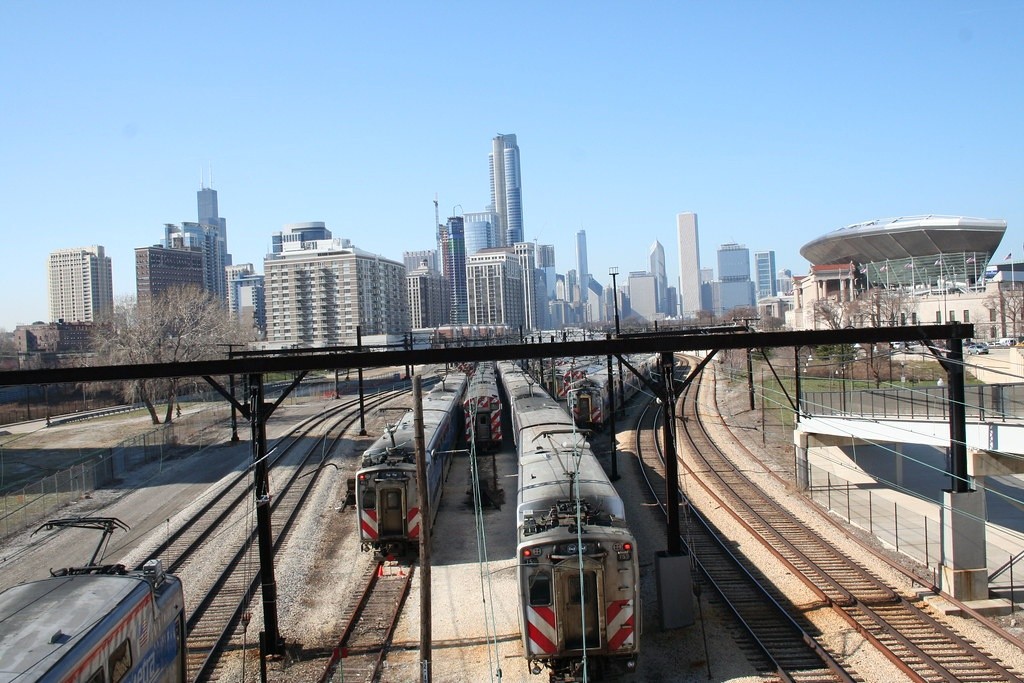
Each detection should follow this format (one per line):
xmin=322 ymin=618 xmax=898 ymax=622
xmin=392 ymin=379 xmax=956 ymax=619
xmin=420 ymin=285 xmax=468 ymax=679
xmin=963 ymin=337 xmax=1024 ymax=347
xmin=968 ymin=345 xmax=988 ymax=355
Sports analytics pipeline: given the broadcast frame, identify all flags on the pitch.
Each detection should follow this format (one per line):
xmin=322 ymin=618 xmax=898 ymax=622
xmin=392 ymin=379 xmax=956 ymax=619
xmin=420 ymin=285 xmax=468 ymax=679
xmin=934 ymin=258 xmax=944 ymax=267
xmin=1003 ymin=254 xmax=1012 ymax=261
xmin=860 ymin=265 xmax=869 ymax=273
xmin=904 ymin=261 xmax=916 ymax=269
xmin=879 ymin=261 xmax=890 ymax=272
xmin=966 ymin=253 xmax=977 ymax=264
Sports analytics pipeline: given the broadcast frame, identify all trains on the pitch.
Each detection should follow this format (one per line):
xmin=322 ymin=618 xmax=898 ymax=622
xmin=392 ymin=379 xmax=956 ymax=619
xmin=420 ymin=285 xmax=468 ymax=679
xmin=496 ymin=360 xmax=643 ymax=683
xmin=461 ymin=361 xmax=505 ymax=454
xmin=351 ymin=363 xmax=474 ymax=563
xmin=1 ymin=516 xmax=190 ymax=682
xmin=527 ymin=354 xmax=661 ymax=433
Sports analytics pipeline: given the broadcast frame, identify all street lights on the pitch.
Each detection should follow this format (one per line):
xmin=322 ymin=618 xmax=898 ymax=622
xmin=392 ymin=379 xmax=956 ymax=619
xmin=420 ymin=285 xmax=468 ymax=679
xmin=840 ymin=325 xmax=861 ymax=418
xmin=608 ymin=266 xmax=621 ymax=338
